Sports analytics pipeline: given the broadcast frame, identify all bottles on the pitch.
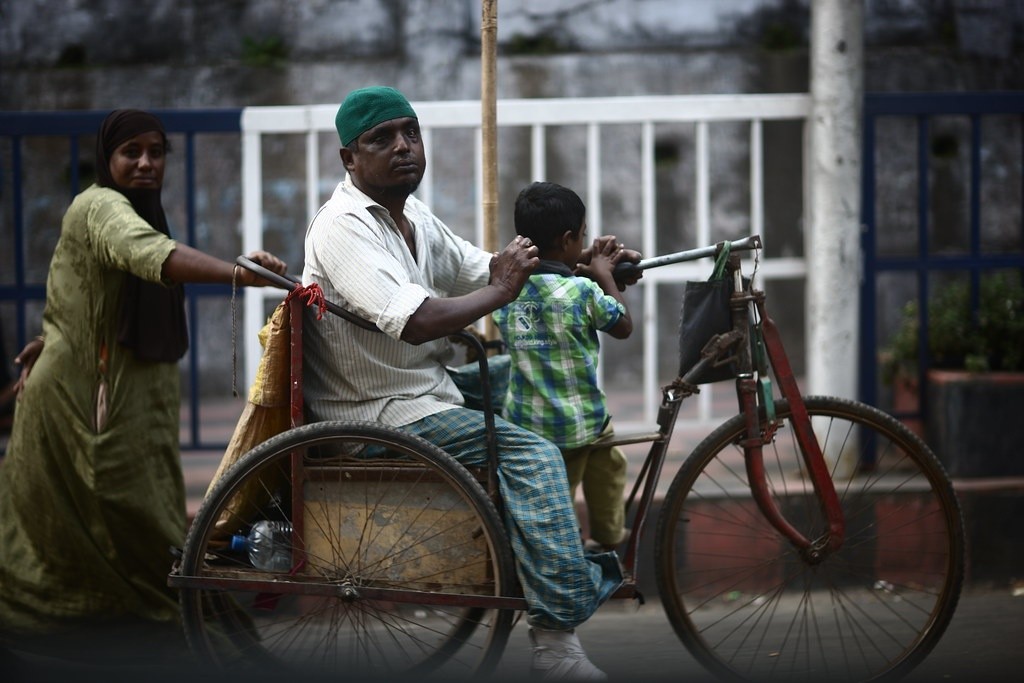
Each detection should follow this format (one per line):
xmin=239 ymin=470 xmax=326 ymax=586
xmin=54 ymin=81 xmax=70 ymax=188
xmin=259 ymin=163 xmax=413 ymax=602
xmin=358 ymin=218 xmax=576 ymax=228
xmin=231 ymin=519 xmax=293 ymax=574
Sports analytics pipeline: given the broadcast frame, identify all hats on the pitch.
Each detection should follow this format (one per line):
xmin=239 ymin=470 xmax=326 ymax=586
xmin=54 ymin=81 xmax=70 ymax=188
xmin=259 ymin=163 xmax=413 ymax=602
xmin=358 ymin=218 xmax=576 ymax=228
xmin=336 ymin=85 xmax=416 ymax=146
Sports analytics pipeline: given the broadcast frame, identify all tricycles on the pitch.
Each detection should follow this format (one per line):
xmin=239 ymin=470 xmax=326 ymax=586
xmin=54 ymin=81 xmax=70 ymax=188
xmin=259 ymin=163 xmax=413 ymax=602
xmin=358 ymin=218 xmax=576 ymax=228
xmin=165 ymin=232 xmax=966 ymax=678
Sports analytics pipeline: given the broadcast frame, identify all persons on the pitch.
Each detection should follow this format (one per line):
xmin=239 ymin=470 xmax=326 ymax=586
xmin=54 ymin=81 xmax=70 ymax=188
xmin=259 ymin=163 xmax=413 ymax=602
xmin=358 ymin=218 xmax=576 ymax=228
xmin=492 ymin=181 xmax=633 ymax=552
xmin=0 ymin=108 xmax=289 ymax=683
xmin=300 ymin=86 xmax=644 ymax=683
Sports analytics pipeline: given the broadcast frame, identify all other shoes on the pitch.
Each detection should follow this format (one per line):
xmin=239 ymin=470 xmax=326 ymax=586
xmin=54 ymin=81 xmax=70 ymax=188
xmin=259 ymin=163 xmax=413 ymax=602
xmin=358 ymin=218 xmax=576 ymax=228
xmin=526 ymin=631 xmax=608 ymax=682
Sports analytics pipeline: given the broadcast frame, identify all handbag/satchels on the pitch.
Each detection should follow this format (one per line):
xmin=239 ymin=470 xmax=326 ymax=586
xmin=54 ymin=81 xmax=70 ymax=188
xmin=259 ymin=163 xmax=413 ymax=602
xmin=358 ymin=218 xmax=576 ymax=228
xmin=678 ymin=245 xmax=770 ymax=386
